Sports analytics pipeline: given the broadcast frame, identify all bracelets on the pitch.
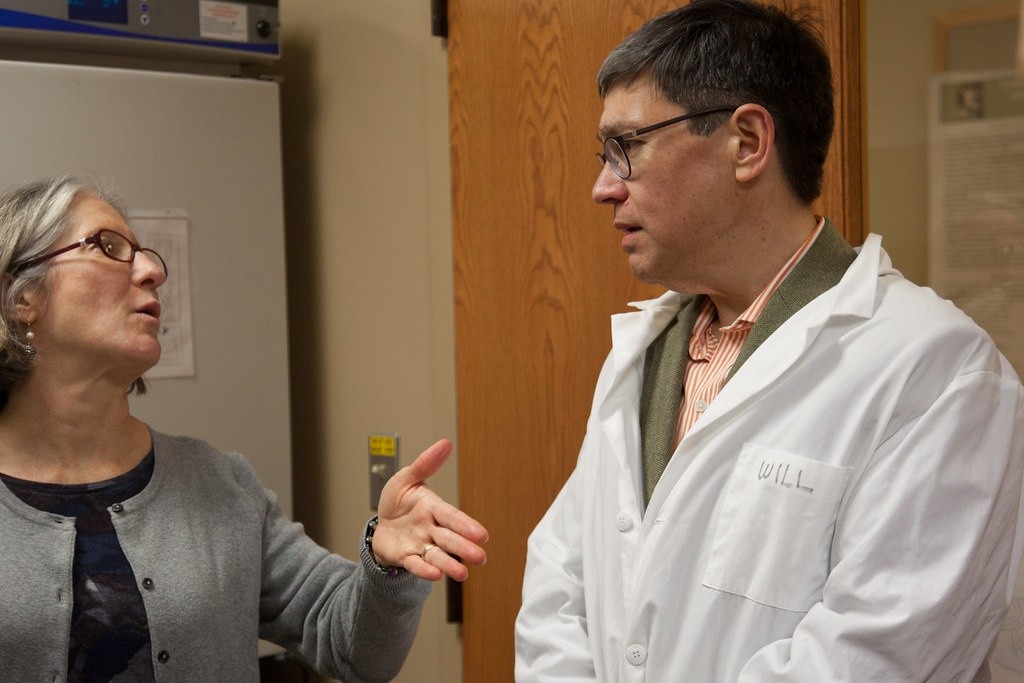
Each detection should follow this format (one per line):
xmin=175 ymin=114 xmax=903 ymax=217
xmin=366 ymin=517 xmax=408 ymax=577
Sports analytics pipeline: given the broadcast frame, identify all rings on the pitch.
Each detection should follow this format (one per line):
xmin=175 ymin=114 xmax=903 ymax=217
xmin=421 ymin=544 xmax=434 ymax=560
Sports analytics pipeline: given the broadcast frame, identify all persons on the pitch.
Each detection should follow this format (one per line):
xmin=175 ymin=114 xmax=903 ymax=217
xmin=513 ymin=0 xmax=1024 ymax=683
xmin=0 ymin=172 xmax=491 ymax=683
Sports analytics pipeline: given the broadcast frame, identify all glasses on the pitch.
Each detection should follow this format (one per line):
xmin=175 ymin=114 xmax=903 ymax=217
xmin=13 ymin=228 xmax=168 ymax=277
xmin=595 ymin=106 xmax=734 ymax=179
